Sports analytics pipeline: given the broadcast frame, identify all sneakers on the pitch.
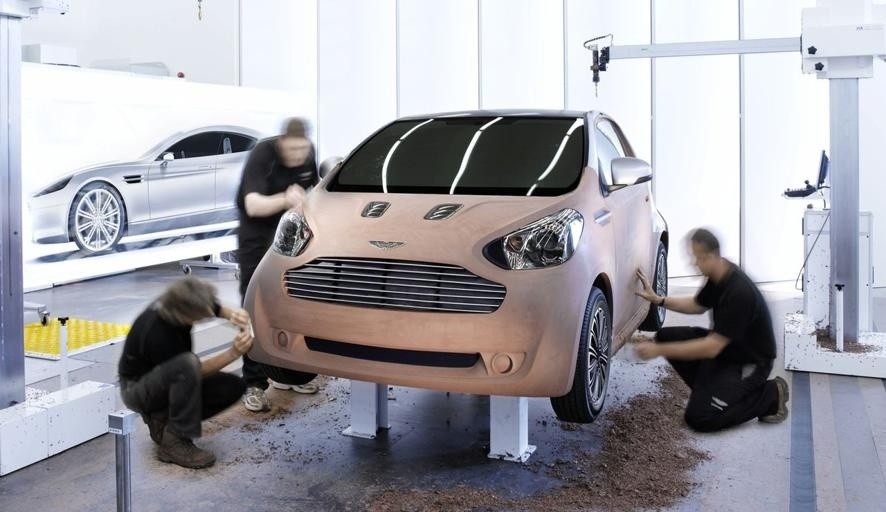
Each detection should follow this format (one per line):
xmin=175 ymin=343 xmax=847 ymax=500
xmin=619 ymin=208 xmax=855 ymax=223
xmin=274 ymin=380 xmax=319 ymax=393
xmin=242 ymin=386 xmax=271 ymax=412
xmin=760 ymin=376 xmax=789 ymax=422
xmin=157 ymin=431 xmax=216 ymax=467
xmin=142 ymin=410 xmax=164 ymax=445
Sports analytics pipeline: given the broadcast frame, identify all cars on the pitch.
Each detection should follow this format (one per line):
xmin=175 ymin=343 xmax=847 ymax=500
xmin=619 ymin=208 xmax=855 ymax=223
xmin=33 ymin=124 xmax=258 ymax=254
xmin=240 ymin=106 xmax=670 ymax=422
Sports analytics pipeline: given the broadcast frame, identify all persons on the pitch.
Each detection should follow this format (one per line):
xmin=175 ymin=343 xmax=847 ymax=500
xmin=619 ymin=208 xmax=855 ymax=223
xmin=632 ymin=229 xmax=790 ymax=434
xmin=119 ymin=275 xmax=253 ymax=472
xmin=236 ymin=117 xmax=322 ymax=413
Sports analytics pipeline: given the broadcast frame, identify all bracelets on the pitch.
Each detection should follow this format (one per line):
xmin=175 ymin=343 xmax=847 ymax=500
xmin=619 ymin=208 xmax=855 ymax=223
xmin=659 ymin=297 xmax=664 ymax=306
